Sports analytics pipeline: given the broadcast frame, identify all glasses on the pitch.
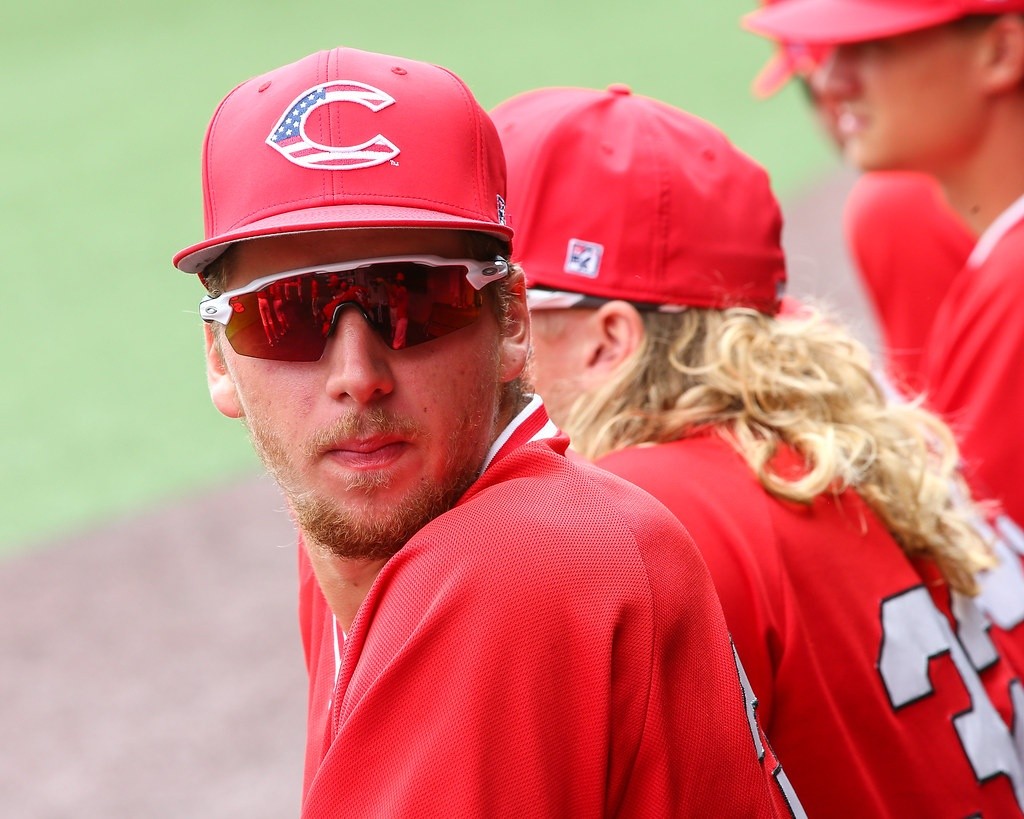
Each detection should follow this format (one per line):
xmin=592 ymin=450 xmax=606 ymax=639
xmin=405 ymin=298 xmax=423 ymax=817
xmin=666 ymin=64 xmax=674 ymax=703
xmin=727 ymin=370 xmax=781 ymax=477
xmin=200 ymin=254 xmax=505 ymax=364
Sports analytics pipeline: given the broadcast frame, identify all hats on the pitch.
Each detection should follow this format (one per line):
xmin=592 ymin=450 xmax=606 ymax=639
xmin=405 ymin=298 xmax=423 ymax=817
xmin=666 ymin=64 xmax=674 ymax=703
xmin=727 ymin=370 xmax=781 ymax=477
xmin=745 ymin=0 xmax=1024 ymax=46
xmin=753 ymin=0 xmax=833 ymax=97
xmin=172 ymin=49 xmax=514 ymax=292
xmin=486 ymin=86 xmax=789 ymax=318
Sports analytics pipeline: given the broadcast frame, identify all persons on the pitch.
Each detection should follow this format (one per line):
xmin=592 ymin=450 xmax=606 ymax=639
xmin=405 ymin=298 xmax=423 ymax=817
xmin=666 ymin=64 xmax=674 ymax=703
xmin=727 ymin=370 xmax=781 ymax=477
xmin=171 ymin=46 xmax=807 ymax=819
xmin=255 ymin=266 xmax=433 ymax=349
xmin=480 ymin=81 xmax=1024 ymax=817
xmin=746 ymin=2 xmax=1024 ymax=557
